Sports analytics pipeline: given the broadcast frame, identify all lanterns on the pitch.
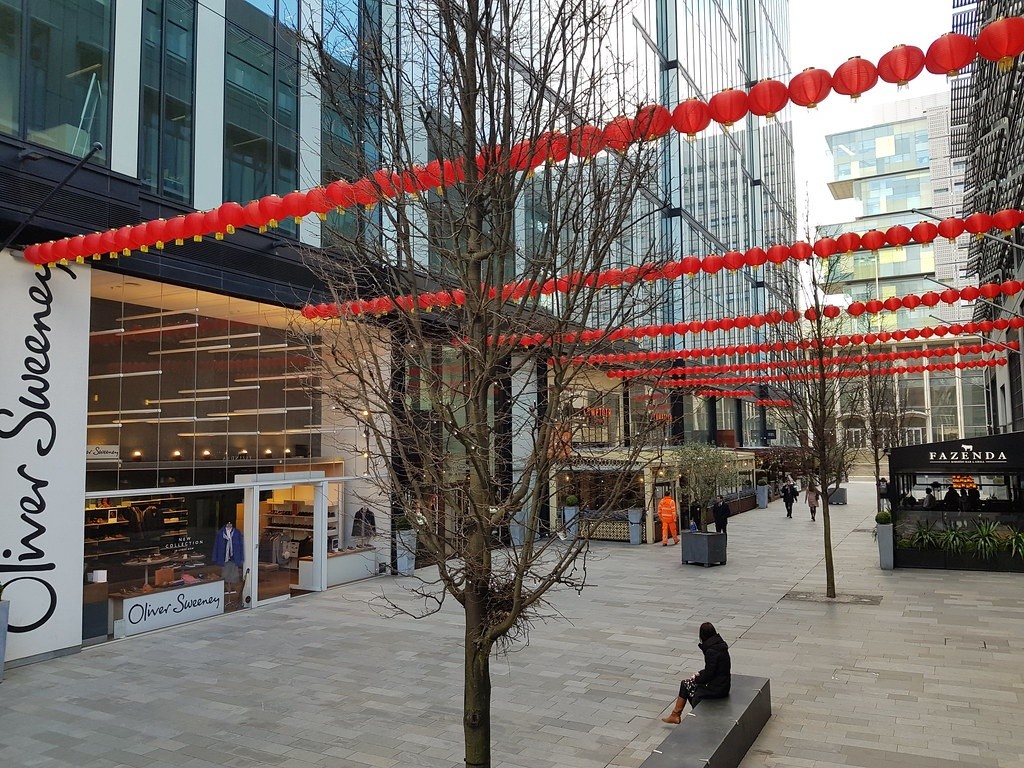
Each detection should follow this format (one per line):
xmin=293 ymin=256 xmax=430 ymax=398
xmin=833 ymin=15 xmax=1024 ymax=103
xmin=605 ymin=241 xmax=812 ymax=286
xmin=453 ymin=280 xmax=1024 ymax=406
xmin=451 ymin=270 xmax=604 ymax=308
xmin=184 ymin=176 xmax=355 ymax=242
xmin=300 ymin=290 xmax=451 ymax=322
xmin=24 ymin=214 xmax=184 ymax=271
xmin=814 ymin=208 xmax=1024 ymax=263
xmin=570 ymin=67 xmax=833 ymax=163
xmin=354 ymin=131 xmax=569 ymax=210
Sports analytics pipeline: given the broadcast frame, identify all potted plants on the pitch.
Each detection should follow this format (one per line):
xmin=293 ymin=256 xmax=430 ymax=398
xmin=676 ymin=442 xmax=736 ymax=565
xmin=757 ymin=479 xmax=769 ymax=509
xmin=895 ymin=516 xmax=1024 ymax=573
xmin=565 ymin=496 xmax=579 ymax=541
xmin=627 ymin=500 xmax=644 ymax=545
xmin=509 ymin=500 xmax=525 ymax=545
xmin=396 ymin=517 xmax=418 ymax=576
xmin=821 ymin=449 xmax=849 ymax=504
xmin=875 ymin=512 xmax=894 ymax=570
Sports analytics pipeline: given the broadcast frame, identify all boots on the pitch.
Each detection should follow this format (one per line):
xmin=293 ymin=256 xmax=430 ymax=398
xmin=662 ymin=695 xmax=686 ymax=725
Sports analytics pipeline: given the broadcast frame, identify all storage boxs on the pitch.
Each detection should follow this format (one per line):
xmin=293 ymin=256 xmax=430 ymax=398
xmin=87 ymin=570 xmax=108 ymax=583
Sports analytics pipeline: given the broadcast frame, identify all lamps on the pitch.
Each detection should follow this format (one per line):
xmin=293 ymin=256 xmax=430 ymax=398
xmin=203 ymin=450 xmax=211 ymax=460
xmin=265 ymin=449 xmax=273 ymax=458
xmin=135 ymin=450 xmax=141 ymax=461
xmin=239 ymin=450 xmax=248 ymax=459
xmin=174 ymin=451 xmax=182 ymax=460
xmin=284 ymin=448 xmax=292 ymax=458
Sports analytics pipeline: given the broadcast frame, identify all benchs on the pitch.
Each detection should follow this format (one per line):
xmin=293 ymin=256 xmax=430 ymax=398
xmin=258 ymin=562 xmax=279 ymax=582
xmin=638 ymin=673 xmax=771 ymax=768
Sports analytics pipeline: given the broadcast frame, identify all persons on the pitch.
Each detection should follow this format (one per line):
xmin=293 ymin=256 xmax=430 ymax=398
xmin=780 ymin=480 xmax=797 ymax=518
xmin=661 ymin=621 xmax=732 ymax=724
xmin=689 ymin=493 xmax=708 ymax=531
xmin=657 ymin=491 xmax=680 ymax=545
xmin=713 ymin=495 xmax=731 ymax=545
xmin=878 ymin=477 xmax=891 ymax=512
xmin=212 ymin=524 xmax=244 ymax=584
xmin=922 ymin=484 xmax=979 ymax=509
xmin=804 ymin=482 xmax=821 ymax=521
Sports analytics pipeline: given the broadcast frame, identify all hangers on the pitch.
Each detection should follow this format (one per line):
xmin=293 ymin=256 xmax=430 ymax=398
xmin=270 ymin=534 xmax=287 ymax=542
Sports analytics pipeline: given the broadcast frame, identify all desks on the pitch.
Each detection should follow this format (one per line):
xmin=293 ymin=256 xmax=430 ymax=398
xmin=298 ymin=545 xmax=377 ymax=586
xmin=108 ymin=575 xmax=225 ymax=637
xmin=123 ymin=557 xmax=170 ymax=586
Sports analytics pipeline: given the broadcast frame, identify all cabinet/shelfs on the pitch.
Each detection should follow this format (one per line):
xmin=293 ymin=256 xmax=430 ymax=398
xmin=262 ymin=510 xmax=339 ymax=534
xmin=84 ymin=497 xmax=190 ymax=560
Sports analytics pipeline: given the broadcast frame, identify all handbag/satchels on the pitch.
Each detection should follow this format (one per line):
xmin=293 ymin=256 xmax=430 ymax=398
xmin=815 ymin=494 xmax=819 ymax=499
